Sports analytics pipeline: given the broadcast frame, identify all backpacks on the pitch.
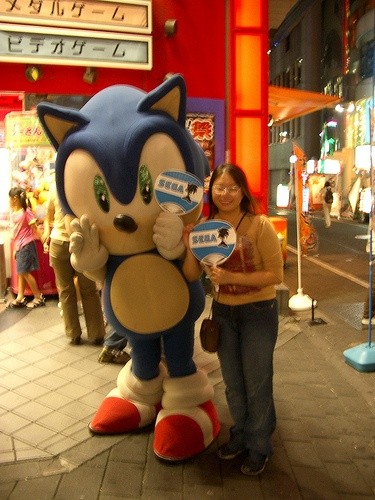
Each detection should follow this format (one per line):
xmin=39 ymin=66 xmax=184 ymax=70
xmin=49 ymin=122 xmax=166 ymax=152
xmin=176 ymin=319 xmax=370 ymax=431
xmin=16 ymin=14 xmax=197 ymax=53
xmin=324 ymin=188 xmax=333 ymax=203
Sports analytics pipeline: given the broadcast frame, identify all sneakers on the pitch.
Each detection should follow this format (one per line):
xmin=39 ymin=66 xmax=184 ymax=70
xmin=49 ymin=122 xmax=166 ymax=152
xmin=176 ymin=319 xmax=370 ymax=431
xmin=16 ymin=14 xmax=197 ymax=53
xmin=98 ymin=346 xmax=131 ymax=364
xmin=240 ymin=450 xmax=270 ymax=475
xmin=219 ymin=443 xmax=244 ymax=460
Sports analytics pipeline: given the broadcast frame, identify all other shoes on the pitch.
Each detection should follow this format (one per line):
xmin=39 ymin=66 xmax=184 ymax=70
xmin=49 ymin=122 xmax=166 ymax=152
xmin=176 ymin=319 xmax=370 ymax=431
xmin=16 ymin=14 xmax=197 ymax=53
xmin=5 ymin=297 xmax=27 ymax=308
xmin=26 ymin=294 xmax=46 ymax=308
xmin=93 ymin=337 xmax=104 ymax=345
xmin=71 ymin=335 xmax=81 ymax=346
xmin=361 ymin=316 xmax=375 ymax=326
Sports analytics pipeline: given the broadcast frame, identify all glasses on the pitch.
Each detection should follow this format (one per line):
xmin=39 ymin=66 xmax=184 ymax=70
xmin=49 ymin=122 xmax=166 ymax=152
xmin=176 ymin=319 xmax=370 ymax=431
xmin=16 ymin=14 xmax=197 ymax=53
xmin=211 ymin=184 xmax=243 ymax=195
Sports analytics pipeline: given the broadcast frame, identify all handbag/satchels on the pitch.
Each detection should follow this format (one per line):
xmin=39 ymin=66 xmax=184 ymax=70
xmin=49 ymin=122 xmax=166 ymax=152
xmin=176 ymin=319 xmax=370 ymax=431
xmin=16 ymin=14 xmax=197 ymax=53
xmin=200 ymin=319 xmax=219 ymax=353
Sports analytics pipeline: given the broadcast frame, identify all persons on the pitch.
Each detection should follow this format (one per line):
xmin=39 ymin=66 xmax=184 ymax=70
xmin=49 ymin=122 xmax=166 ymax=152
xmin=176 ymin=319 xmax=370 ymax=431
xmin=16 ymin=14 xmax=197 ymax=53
xmin=321 ymin=182 xmax=334 ymax=228
xmin=182 ymin=163 xmax=284 ymax=476
xmin=8 ymin=188 xmax=48 ymax=309
xmin=97 ymin=325 xmax=131 ymax=366
xmin=361 ymin=204 xmax=375 ymax=325
xmin=42 ymin=182 xmax=106 ymax=346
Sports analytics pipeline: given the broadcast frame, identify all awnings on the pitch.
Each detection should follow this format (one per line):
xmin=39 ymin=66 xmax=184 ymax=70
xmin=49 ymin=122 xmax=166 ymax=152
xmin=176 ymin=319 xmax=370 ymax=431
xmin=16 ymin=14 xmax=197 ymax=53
xmin=268 ymin=85 xmax=342 ymax=128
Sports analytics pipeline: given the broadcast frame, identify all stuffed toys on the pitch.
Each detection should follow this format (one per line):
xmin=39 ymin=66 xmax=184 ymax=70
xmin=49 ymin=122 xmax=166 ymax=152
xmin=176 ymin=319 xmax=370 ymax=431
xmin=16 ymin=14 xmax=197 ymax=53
xmin=12 ymin=148 xmax=55 ymax=212
xmin=35 ymin=75 xmax=220 ymax=460
